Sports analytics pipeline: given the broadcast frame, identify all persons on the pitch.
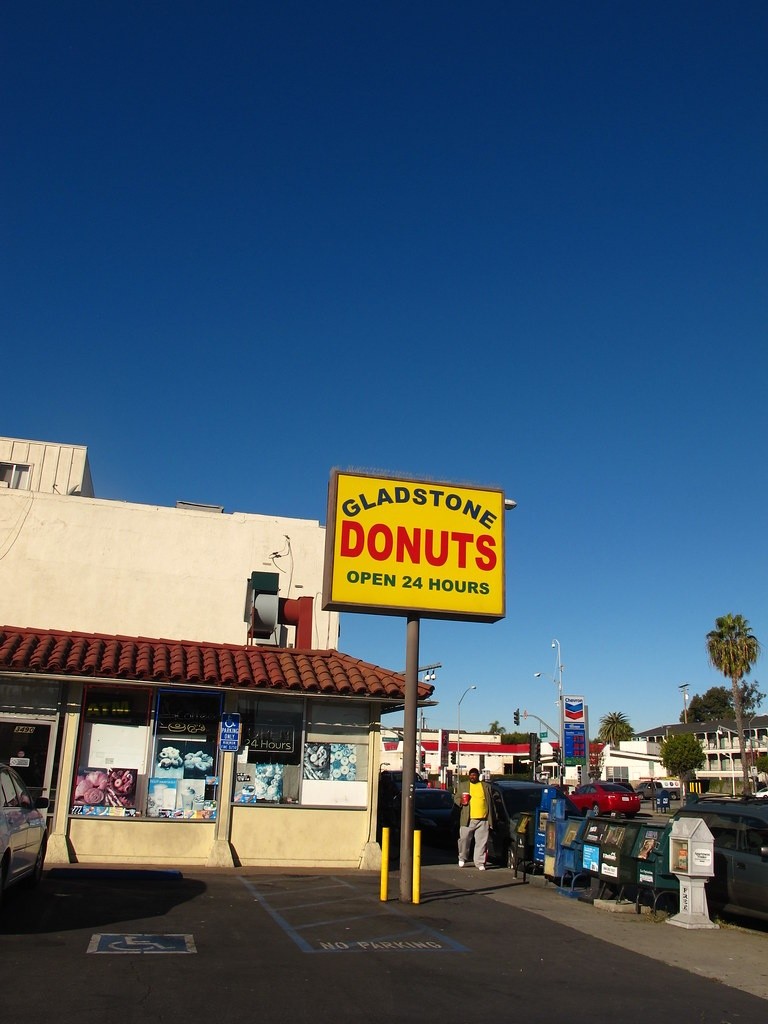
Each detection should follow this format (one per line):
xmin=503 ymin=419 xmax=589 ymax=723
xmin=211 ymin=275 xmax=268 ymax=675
xmin=454 ymin=767 xmax=493 ymax=870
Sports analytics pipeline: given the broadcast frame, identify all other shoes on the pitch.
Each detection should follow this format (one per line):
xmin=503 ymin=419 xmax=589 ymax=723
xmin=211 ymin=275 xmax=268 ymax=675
xmin=458 ymin=861 xmax=465 ymax=867
xmin=478 ymin=866 xmax=485 ymax=870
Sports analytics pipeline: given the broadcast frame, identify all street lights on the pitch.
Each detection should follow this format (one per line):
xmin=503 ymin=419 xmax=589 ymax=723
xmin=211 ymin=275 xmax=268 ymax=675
xmin=722 ymin=752 xmax=736 ymax=797
xmin=534 ymin=638 xmax=565 ymax=779
xmin=457 ymin=684 xmax=477 ymax=789
xmin=375 ymin=708 xmax=424 ymax=781
xmin=379 ymin=762 xmax=389 ymax=774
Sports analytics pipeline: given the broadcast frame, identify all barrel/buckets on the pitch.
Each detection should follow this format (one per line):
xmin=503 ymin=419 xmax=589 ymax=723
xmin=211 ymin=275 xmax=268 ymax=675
xmin=306 ymin=749 xmax=329 ymax=771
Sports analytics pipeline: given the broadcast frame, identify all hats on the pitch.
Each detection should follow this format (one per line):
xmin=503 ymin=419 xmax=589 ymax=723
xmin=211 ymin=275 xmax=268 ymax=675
xmin=469 ymin=768 xmax=478 ymax=774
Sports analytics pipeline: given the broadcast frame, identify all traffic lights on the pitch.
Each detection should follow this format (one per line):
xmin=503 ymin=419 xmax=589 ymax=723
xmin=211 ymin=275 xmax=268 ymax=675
xmin=513 ymin=708 xmax=519 ymax=726
xmin=552 ymin=748 xmax=560 ymax=764
xmin=532 ymin=742 xmax=540 ymax=762
xmin=451 ymin=750 xmax=456 ymax=764
xmin=422 ymin=767 xmax=425 ymax=772
xmin=420 ymin=751 xmax=425 ymax=764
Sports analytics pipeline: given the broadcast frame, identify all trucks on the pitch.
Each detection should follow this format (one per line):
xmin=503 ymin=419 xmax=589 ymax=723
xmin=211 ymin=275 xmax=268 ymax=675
xmin=635 ymin=781 xmax=679 ymax=800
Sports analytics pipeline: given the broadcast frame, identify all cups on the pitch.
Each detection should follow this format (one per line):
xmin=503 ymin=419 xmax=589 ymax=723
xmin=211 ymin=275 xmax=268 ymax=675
xmin=462 ymin=792 xmax=469 ymax=805
xmin=181 ymin=794 xmax=204 ymax=810
xmin=153 ymin=784 xmax=176 ymax=811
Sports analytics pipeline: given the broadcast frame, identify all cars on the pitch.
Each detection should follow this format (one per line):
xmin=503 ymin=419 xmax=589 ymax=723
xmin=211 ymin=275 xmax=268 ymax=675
xmin=751 ymin=787 xmax=768 ymax=799
xmin=1 ymin=761 xmax=51 ymax=895
xmin=670 ymin=793 xmax=768 ymax=926
xmin=394 ymin=788 xmax=460 ymax=845
xmin=568 ymin=784 xmax=640 ymax=819
xmin=380 ymin=771 xmax=427 ymax=803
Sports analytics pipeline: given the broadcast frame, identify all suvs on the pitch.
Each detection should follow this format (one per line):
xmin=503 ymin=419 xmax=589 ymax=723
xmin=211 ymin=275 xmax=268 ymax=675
xmin=483 ymin=777 xmax=581 ymax=864
